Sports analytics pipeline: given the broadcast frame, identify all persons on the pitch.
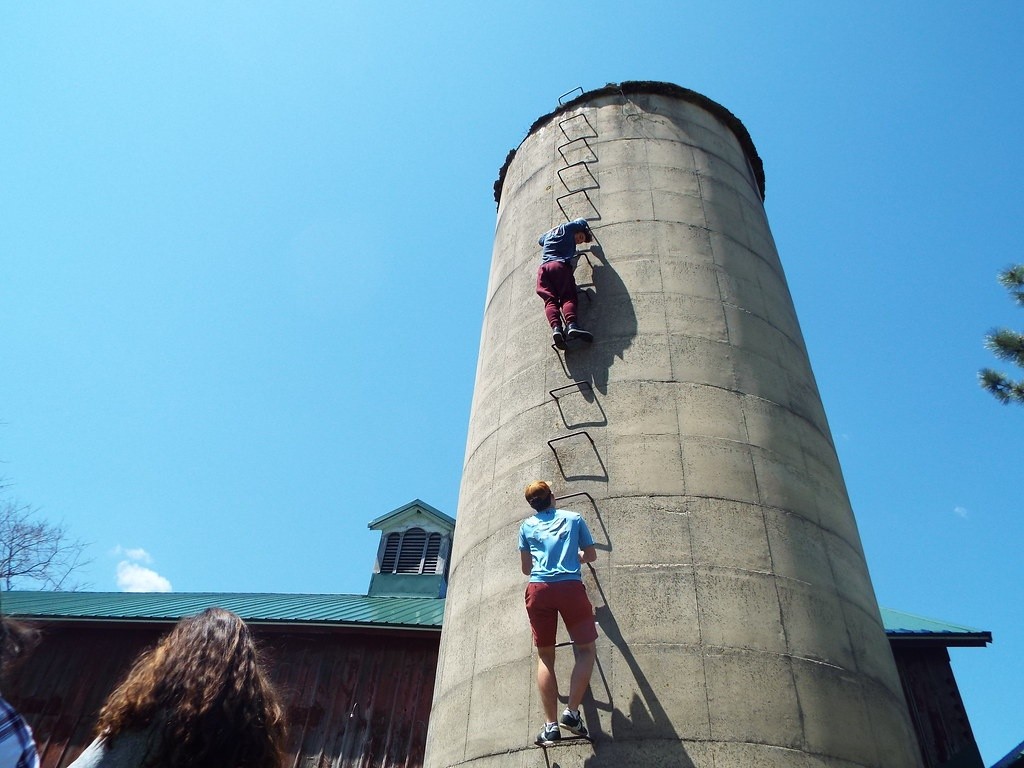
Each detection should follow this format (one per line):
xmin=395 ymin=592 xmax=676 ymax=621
xmin=66 ymin=608 xmax=290 ymax=768
xmin=0 ymin=617 xmax=46 ymax=768
xmin=517 ymin=480 xmax=598 ymax=745
xmin=537 ymin=218 xmax=593 ymax=349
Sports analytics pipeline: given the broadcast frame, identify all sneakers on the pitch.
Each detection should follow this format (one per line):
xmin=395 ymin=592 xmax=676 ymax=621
xmin=534 ymin=722 xmax=561 ymax=745
xmin=559 ymin=708 xmax=587 ymax=735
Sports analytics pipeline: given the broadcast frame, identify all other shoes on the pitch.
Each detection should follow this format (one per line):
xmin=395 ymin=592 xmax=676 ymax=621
xmin=567 ymin=323 xmax=593 ymax=343
xmin=552 ymin=327 xmax=568 ymax=350
